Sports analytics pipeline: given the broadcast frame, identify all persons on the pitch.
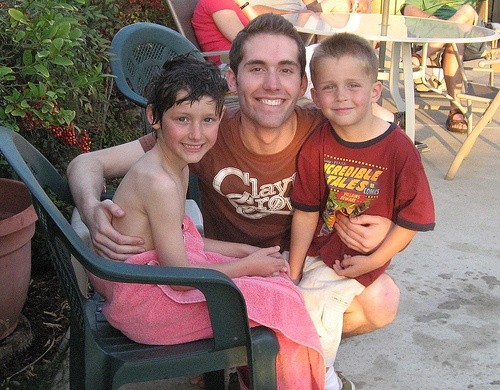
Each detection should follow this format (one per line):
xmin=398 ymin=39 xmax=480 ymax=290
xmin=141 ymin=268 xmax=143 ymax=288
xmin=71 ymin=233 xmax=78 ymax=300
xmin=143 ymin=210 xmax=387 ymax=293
xmin=400 ymin=0 xmax=491 ymax=134
xmin=89 ymin=50 xmax=326 ymax=390
xmin=191 ymin=1 xmax=430 ymax=153
xmin=281 ymin=31 xmax=436 ymax=390
xmin=280 ymin=12 xmax=350 ymax=32
xmin=405 ymin=17 xmax=473 ymax=38
xmin=66 ymin=13 xmax=412 ymax=387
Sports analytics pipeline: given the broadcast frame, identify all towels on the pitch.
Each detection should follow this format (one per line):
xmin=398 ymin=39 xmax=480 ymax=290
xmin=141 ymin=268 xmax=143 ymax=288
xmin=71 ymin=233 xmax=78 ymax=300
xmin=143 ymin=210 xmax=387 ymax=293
xmin=85 ymin=215 xmax=325 ymax=389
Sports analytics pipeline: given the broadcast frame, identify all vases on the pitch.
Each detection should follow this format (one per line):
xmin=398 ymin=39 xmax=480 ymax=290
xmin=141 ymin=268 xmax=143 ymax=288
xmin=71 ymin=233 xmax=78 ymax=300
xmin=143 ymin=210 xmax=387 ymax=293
xmin=0 ymin=178 xmax=38 ymax=343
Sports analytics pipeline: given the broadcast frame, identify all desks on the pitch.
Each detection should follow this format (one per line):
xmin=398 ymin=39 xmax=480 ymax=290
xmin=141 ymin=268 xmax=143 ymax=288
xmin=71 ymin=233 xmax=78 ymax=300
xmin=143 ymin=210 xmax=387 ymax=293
xmin=278 ymin=11 xmax=498 ymax=150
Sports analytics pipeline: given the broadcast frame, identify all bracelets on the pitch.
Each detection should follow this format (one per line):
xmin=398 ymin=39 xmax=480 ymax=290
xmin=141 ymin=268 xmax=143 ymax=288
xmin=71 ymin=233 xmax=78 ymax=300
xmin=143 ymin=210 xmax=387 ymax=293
xmin=240 ymin=2 xmax=249 ymax=10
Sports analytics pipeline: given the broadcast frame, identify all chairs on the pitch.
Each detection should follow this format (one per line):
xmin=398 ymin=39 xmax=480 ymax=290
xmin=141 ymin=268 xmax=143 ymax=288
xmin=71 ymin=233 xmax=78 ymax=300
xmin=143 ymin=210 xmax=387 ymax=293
xmin=0 ymin=125 xmax=281 ymax=390
xmin=109 ymin=0 xmax=500 ymax=181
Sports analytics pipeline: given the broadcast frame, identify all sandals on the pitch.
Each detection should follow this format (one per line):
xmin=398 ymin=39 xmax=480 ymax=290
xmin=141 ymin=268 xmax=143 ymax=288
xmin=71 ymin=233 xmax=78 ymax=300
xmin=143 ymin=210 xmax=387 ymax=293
xmin=446 ymin=107 xmax=468 ymax=134
